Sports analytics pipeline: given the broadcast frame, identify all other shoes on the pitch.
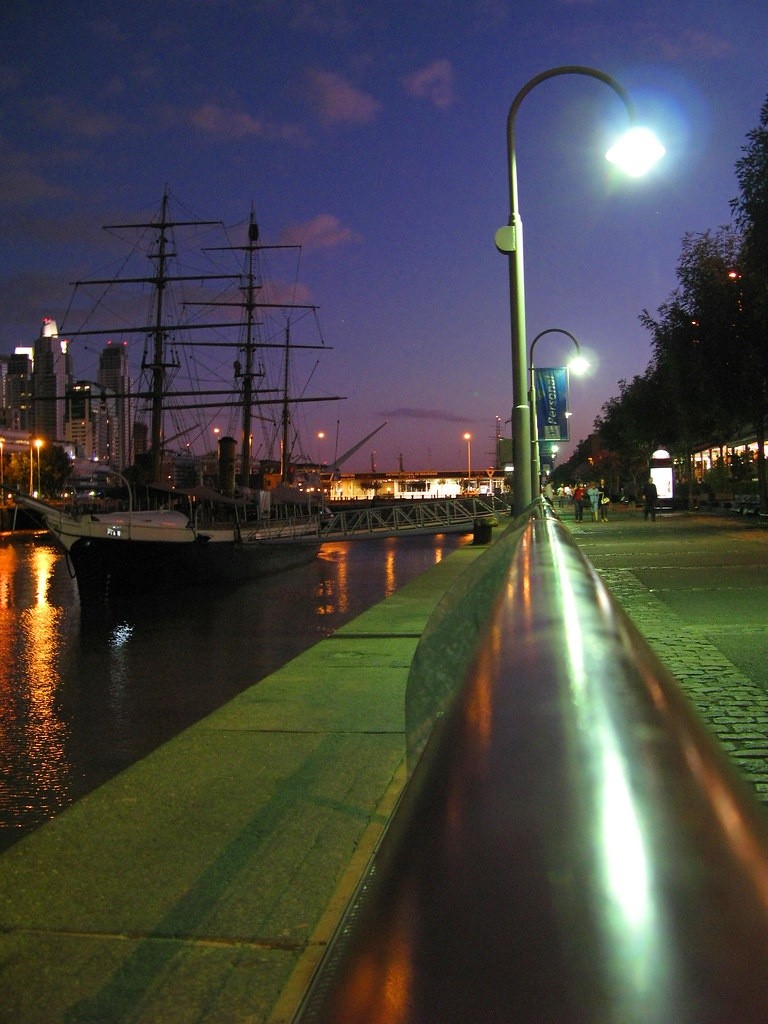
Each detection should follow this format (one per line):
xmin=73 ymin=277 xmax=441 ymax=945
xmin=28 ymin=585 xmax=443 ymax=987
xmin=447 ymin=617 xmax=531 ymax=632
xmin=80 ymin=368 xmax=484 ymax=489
xmin=602 ymin=518 xmax=604 ymax=522
xmin=605 ymin=518 xmax=608 ymax=521
xmin=576 ymin=519 xmax=583 ymax=523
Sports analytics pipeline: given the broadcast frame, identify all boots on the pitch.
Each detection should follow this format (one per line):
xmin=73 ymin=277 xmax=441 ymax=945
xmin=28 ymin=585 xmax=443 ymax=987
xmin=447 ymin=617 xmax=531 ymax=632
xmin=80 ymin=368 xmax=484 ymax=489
xmin=591 ymin=511 xmax=594 ymax=522
xmin=595 ymin=511 xmax=599 ymax=522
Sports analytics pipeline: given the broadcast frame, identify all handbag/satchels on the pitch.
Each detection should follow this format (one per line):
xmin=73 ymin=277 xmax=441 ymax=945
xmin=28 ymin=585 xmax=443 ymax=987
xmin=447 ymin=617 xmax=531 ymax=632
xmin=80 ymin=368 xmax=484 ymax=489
xmin=601 ymin=493 xmax=610 ymax=505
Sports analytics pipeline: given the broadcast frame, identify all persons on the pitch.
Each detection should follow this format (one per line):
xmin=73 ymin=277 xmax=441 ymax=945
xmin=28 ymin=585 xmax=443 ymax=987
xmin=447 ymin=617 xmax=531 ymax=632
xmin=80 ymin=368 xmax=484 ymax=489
xmin=573 ymin=479 xmax=612 ymax=524
xmin=641 ymin=477 xmax=658 ymax=523
xmin=557 ymin=483 xmax=573 ymax=510
xmin=545 ymin=479 xmax=555 ymax=508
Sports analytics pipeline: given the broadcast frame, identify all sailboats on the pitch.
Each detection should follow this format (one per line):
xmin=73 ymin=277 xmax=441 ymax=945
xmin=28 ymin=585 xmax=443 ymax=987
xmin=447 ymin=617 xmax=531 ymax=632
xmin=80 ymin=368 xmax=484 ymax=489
xmin=0 ymin=182 xmax=348 ymax=622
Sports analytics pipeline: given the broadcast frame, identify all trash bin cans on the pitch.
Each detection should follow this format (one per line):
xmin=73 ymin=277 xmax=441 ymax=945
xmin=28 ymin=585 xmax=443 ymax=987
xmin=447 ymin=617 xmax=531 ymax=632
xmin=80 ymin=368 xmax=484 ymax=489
xmin=473 ymin=518 xmax=492 ymax=544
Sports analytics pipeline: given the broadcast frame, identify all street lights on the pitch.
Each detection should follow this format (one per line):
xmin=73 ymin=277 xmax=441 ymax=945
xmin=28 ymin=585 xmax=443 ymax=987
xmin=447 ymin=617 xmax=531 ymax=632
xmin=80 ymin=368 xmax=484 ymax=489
xmin=529 ymin=328 xmax=590 ymax=499
xmin=506 ymin=63 xmax=664 ymax=515
xmin=34 ymin=439 xmax=45 ymax=499
xmin=464 ymin=433 xmax=471 ymax=492
